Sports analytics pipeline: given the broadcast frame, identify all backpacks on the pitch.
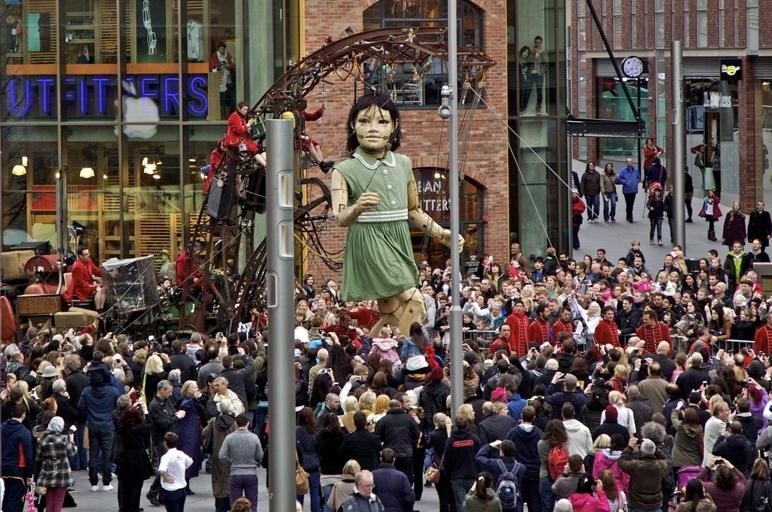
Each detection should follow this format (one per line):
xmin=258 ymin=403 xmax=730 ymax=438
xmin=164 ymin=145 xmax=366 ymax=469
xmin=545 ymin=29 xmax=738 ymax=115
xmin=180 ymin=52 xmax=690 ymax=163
xmin=495 ymin=459 xmax=520 ymax=509
xmin=548 ymin=444 xmax=568 ymax=481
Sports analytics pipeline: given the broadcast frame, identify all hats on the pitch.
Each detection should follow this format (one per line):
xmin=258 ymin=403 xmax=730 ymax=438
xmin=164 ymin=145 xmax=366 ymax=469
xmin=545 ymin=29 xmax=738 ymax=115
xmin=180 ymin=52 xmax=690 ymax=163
xmin=565 ymin=374 xmax=577 ymax=385
xmin=48 ymin=416 xmax=64 ymax=432
xmin=740 ymin=280 xmax=753 ymax=287
xmin=606 ymin=405 xmax=618 ymax=417
xmin=501 ymin=440 xmax=515 ymax=455
xmin=41 ymin=366 xmax=58 ymax=378
xmin=206 ymin=373 xmax=218 ymax=383
xmin=580 ymin=473 xmax=597 ymax=486
xmin=431 ymin=367 xmax=444 ymax=382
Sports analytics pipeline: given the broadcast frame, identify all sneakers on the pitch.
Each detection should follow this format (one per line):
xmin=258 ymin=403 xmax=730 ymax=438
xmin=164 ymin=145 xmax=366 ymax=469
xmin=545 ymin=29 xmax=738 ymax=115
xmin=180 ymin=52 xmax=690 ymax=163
xmin=187 ymin=490 xmax=196 ymax=496
xmin=685 ymin=219 xmax=692 ymax=223
xmin=424 ymin=482 xmax=431 ymax=488
xmin=322 ymin=160 xmax=335 ymax=174
xmin=91 ymin=485 xmax=114 ymax=491
xmin=626 ymin=217 xmax=633 ymax=223
xmin=588 ymin=219 xmax=617 ymax=223
xmin=650 ymin=240 xmax=664 ymax=246
xmin=146 ymin=492 xmax=160 ymax=506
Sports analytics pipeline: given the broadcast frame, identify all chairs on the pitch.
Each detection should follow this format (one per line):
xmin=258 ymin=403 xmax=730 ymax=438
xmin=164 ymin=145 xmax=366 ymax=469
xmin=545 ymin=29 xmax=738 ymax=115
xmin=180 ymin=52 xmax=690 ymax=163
xmin=63 ymin=271 xmax=95 ymax=308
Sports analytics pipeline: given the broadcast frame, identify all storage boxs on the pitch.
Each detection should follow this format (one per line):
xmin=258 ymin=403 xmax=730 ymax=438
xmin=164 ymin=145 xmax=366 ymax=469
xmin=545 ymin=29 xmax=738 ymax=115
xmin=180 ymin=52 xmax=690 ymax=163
xmin=16 ymin=293 xmax=62 ymax=315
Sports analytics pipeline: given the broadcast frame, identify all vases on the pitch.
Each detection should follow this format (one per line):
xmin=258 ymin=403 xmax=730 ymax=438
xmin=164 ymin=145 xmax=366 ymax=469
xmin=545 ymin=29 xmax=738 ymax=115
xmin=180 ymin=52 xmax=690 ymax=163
xmin=6 ymin=52 xmax=23 ymax=64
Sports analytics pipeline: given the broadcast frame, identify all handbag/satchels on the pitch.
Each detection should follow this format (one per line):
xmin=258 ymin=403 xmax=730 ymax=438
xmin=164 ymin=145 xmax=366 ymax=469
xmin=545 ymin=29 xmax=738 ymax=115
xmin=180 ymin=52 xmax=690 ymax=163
xmin=649 ymin=181 xmax=662 ymax=194
xmin=202 ymin=430 xmax=213 ymax=453
xmin=296 ymin=465 xmax=309 ymax=496
xmin=694 ymin=153 xmax=704 ymax=169
xmin=755 ymin=496 xmax=770 ymax=512
xmin=615 ymin=195 xmax=618 ymax=202
xmin=327 ymin=487 xmax=335 ymax=510
xmin=424 ymin=465 xmax=440 ymax=484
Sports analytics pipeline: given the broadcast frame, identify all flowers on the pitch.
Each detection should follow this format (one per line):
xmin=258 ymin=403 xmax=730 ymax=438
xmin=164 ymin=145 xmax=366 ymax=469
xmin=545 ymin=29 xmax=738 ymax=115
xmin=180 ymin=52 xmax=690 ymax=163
xmin=6 ymin=15 xmax=22 ymax=53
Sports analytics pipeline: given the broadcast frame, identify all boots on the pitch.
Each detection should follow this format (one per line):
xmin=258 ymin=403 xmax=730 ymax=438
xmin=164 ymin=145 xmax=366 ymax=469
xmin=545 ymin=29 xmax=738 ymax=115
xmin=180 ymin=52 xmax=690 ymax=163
xmin=708 ymin=232 xmax=717 ymax=241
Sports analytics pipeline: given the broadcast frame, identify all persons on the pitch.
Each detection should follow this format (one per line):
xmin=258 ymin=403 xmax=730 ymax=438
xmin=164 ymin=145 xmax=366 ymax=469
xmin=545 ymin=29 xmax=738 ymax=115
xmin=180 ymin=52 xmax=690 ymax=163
xmin=291 ymin=97 xmax=336 ymax=175
xmin=529 ymin=36 xmax=546 ymax=116
xmin=330 ymin=93 xmax=469 ymax=341
xmin=73 ymin=45 xmax=97 ymax=63
xmin=225 ymin=100 xmax=268 ymax=170
xmin=0 ymin=238 xmax=771 ymax=511
xmin=201 ymin=138 xmax=230 ymax=196
xmin=208 ymin=41 xmax=236 ymax=122
xmin=519 ymin=45 xmax=531 ymax=112
xmin=570 ymin=134 xmax=772 ymax=237
